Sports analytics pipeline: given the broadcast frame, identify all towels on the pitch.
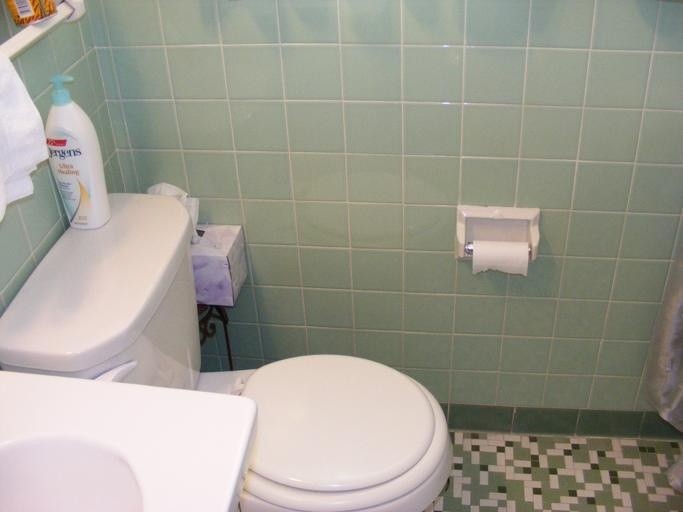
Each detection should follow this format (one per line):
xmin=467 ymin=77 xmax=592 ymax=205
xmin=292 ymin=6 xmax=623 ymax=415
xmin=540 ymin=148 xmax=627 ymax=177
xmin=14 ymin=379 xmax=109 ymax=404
xmin=0 ymin=45 xmax=49 ymax=222
